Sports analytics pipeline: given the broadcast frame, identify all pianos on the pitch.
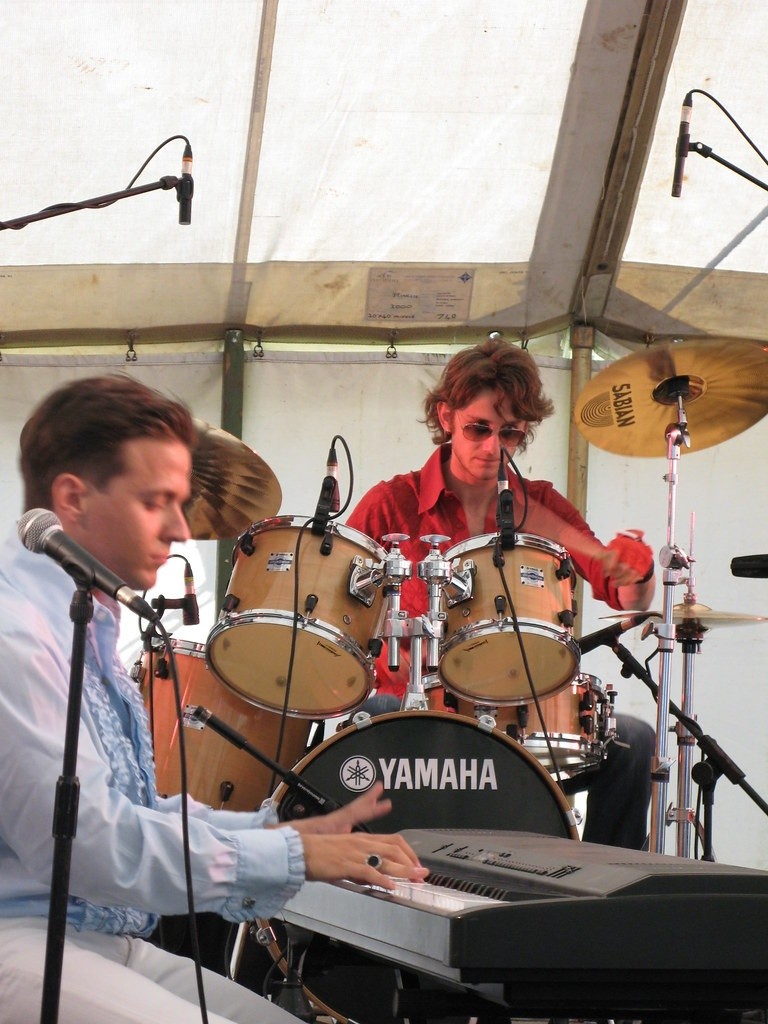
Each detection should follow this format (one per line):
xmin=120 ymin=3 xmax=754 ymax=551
xmin=271 ymin=829 xmax=767 ymax=1024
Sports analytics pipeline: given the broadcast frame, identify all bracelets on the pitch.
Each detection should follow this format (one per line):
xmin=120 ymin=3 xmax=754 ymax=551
xmin=636 ymin=560 xmax=654 ymax=584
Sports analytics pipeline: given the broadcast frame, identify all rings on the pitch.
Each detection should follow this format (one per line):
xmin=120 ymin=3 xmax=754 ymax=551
xmin=365 ymin=853 xmax=382 ymax=870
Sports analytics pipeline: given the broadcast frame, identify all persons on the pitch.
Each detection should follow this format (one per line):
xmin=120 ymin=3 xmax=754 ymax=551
xmin=344 ymin=336 xmax=656 ymax=854
xmin=0 ymin=374 xmax=430 ymax=1024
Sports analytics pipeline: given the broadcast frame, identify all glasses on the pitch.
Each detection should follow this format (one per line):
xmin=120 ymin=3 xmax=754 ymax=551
xmin=453 ymin=407 xmax=526 ymax=447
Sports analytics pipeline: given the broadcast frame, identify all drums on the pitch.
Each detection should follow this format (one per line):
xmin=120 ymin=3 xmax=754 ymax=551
xmin=442 ymin=531 xmax=583 ymax=707
xmin=132 ymin=633 xmax=315 ymax=817
xmin=254 ymin=708 xmax=583 ymax=1024
xmin=423 ymin=667 xmax=618 ymax=767
xmin=205 ymin=512 xmax=390 ymax=720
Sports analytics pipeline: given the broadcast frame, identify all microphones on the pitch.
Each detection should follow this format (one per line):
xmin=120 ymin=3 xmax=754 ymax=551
xmin=326 ymin=448 xmax=340 ymax=512
xmin=672 ymin=93 xmax=692 ymax=197
xmin=495 ymin=462 xmax=509 ymax=528
xmin=576 ymin=613 xmax=653 ymax=655
xmin=183 ymin=562 xmax=200 ymax=626
xmin=179 ymin=147 xmax=193 ymax=225
xmin=17 ymin=507 xmax=159 ymax=625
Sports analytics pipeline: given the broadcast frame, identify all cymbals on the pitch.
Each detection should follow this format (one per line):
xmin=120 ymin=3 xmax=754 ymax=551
xmin=572 ymin=338 xmax=768 ymax=457
xmin=596 ymin=603 xmax=767 ymax=629
xmin=181 ymin=417 xmax=282 ymax=540
